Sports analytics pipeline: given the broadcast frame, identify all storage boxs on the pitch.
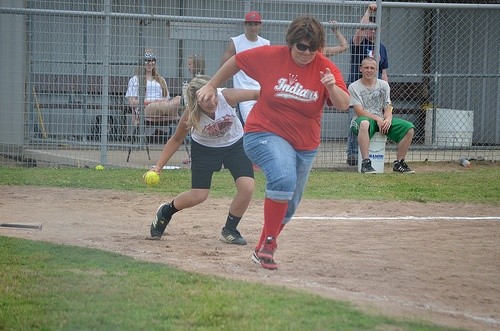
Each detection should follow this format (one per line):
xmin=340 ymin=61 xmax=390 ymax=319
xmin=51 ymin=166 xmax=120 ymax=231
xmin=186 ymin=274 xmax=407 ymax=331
xmin=424 ymin=108 xmax=473 ymax=148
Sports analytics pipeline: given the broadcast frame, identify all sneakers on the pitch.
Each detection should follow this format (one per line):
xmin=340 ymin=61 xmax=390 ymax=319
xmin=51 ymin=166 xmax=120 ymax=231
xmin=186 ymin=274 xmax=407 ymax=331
xmin=149 ymin=203 xmax=171 ymax=238
xmin=250 ymin=235 xmax=278 ymax=269
xmin=219 ymin=226 xmax=247 ymax=245
xmin=392 ymin=159 xmax=415 ymax=174
xmin=361 ymin=159 xmax=376 ymax=174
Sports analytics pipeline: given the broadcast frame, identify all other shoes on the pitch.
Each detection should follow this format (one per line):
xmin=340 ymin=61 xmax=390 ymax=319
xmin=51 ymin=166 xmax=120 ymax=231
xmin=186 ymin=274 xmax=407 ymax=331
xmin=347 ymin=153 xmax=358 ymax=165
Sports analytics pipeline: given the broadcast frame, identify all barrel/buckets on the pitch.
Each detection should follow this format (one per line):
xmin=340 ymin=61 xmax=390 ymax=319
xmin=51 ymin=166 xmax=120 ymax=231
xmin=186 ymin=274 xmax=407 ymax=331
xmin=357 ymin=130 xmax=387 ymax=174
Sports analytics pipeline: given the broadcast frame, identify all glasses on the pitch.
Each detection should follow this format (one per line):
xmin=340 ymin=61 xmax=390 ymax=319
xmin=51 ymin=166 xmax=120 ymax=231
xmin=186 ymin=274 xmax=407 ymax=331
xmin=293 ymin=39 xmax=320 ymax=52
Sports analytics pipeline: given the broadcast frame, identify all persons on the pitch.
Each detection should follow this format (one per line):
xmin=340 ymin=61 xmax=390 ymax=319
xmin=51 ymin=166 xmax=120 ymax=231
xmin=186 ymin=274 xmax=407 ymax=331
xmin=194 ymin=15 xmax=352 ymax=271
xmin=125 ymin=49 xmax=180 ymax=119
xmin=220 ymin=12 xmax=273 ymax=171
xmin=141 ymin=74 xmax=260 ymax=246
xmin=347 ymin=58 xmax=416 ymax=175
xmin=182 ymin=54 xmax=207 ymax=164
xmin=344 ymin=3 xmax=389 ymax=166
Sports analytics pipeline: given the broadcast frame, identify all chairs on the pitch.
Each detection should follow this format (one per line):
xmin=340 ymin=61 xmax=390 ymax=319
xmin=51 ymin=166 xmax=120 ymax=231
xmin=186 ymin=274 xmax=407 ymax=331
xmin=127 ymin=117 xmax=191 ymax=162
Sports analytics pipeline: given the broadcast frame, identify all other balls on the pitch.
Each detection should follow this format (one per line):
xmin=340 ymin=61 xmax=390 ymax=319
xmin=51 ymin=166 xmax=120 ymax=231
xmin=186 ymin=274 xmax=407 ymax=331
xmin=145 ymin=171 xmax=159 ymax=187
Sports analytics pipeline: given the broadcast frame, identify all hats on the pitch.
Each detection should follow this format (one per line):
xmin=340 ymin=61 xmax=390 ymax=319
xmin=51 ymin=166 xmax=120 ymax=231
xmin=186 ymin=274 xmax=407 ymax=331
xmin=243 ymin=12 xmax=263 ymax=23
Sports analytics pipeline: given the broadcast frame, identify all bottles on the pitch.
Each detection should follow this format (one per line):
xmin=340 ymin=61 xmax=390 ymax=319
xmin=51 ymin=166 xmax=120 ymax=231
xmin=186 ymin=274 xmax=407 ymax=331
xmin=458 ymin=157 xmax=471 ymax=168
